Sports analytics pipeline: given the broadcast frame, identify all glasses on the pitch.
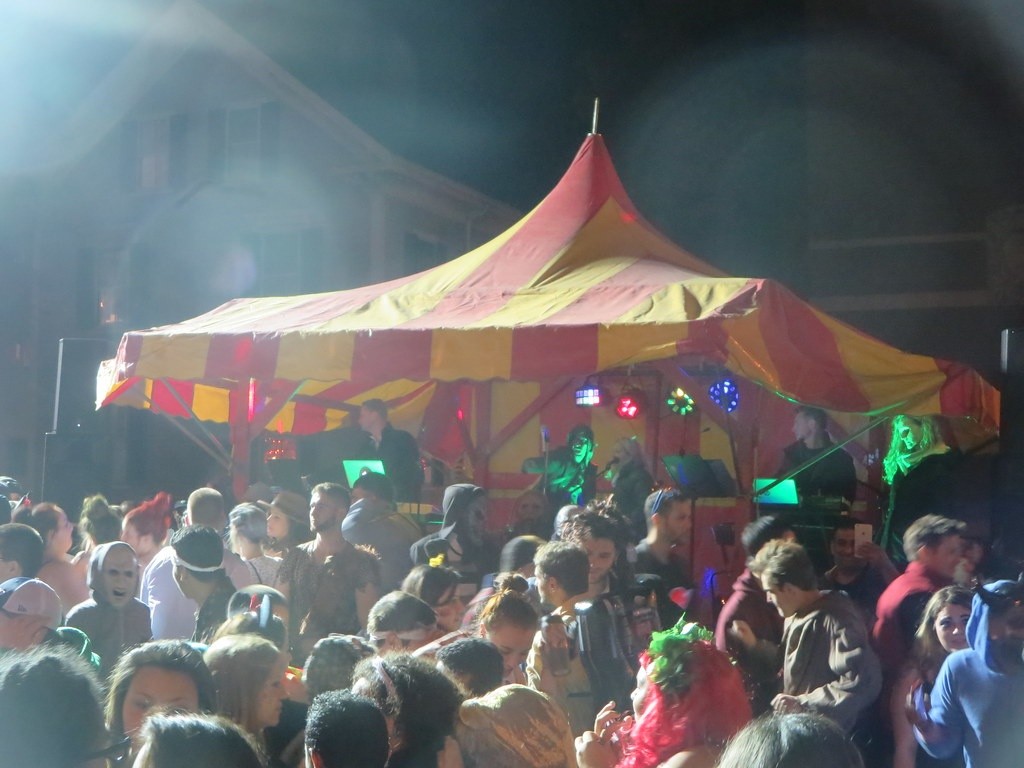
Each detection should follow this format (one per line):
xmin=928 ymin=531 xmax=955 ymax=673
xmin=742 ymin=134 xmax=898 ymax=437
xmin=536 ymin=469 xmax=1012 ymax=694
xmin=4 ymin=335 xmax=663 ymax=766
xmin=652 ymin=487 xmax=682 ymax=514
xmin=60 ymin=731 xmax=131 ymax=768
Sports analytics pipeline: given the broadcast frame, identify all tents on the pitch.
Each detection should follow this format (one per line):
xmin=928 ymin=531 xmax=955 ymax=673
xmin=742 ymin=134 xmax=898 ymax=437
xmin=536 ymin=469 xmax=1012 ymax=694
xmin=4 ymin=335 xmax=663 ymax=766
xmin=93 ymin=133 xmax=1002 ymax=508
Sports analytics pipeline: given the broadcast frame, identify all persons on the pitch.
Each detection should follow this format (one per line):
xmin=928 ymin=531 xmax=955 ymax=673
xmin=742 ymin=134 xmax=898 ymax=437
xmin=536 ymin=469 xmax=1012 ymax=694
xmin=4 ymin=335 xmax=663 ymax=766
xmin=610 ymin=437 xmax=654 ymax=508
xmin=775 ymin=407 xmax=857 ymax=504
xmin=883 ymin=414 xmax=981 ymax=563
xmin=524 ymin=424 xmax=597 ymax=506
xmin=347 ymin=400 xmax=424 ymax=502
xmin=0 ymin=473 xmax=1024 ymax=768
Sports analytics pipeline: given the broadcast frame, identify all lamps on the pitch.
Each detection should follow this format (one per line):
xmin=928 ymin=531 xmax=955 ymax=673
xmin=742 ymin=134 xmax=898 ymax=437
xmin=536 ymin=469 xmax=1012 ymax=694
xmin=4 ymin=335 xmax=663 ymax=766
xmin=615 ymin=375 xmax=649 ymax=419
xmin=575 ymin=375 xmax=613 ymax=408
xmin=709 ymin=378 xmax=739 ymax=414
xmin=665 ymin=385 xmax=696 ymax=417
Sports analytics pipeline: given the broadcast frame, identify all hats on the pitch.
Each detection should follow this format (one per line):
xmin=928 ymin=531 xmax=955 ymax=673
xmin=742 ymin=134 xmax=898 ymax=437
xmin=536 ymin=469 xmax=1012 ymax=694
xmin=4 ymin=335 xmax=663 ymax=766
xmin=256 ymin=491 xmax=308 ymax=525
xmin=0 ymin=577 xmax=62 ymax=625
xmin=499 ymin=534 xmax=547 ymax=572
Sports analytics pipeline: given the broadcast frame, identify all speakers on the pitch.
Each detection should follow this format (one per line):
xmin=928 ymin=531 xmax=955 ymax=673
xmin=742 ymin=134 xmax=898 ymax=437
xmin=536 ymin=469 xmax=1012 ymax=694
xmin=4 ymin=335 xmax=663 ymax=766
xmin=53 ymin=338 xmax=117 ymax=435
xmin=42 ymin=432 xmax=119 ymax=525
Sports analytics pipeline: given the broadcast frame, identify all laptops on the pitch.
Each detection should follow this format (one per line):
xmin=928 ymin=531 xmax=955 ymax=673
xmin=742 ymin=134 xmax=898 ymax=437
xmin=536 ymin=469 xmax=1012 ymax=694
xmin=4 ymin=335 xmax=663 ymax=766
xmin=752 ymin=477 xmax=799 ymax=510
xmin=341 ymin=459 xmax=386 ymax=489
xmin=660 ymin=453 xmax=722 ymax=498
xmin=266 ymin=459 xmax=305 ymax=496
xmin=704 ymin=459 xmax=736 ymax=497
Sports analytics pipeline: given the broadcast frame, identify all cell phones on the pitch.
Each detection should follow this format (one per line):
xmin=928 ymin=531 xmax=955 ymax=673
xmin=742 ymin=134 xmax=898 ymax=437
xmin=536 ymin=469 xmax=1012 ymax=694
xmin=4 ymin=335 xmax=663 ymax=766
xmin=855 ymin=524 xmax=872 ymax=559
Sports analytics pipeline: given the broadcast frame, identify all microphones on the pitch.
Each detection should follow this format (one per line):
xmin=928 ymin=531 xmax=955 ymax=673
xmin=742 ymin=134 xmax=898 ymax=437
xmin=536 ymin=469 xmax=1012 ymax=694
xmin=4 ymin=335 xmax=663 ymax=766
xmin=541 ymin=424 xmax=549 ymax=451
xmin=678 ymin=427 xmax=710 ymax=456
xmin=605 ymin=456 xmax=619 ymax=471
